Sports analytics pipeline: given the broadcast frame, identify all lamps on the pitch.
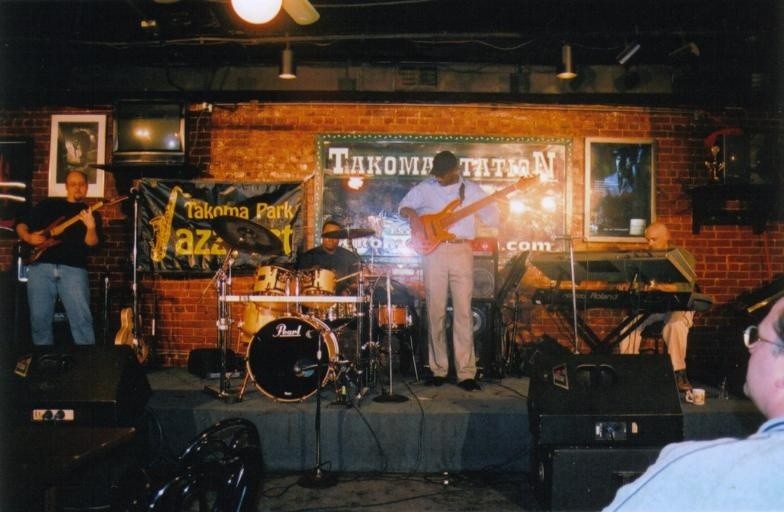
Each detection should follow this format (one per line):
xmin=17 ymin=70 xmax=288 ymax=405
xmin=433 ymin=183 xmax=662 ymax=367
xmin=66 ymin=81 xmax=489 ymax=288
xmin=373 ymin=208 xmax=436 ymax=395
xmin=615 ymin=32 xmax=641 ymax=65
xmin=555 ymin=42 xmax=578 ymax=80
xmin=230 ymin=0 xmax=283 ymax=25
xmin=278 ymin=42 xmax=297 ymax=80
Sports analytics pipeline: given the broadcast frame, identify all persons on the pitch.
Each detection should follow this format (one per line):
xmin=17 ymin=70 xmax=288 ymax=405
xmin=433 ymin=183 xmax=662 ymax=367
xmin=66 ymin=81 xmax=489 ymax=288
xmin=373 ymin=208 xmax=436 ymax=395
xmin=600 ymin=146 xmax=635 ymax=196
xmin=17 ymin=170 xmax=102 ymax=345
xmin=398 ymin=151 xmax=507 ymax=395
xmin=601 ymin=283 xmax=784 ymax=512
xmin=296 ymin=220 xmax=370 ymax=295
xmin=618 ymin=221 xmax=695 ymax=391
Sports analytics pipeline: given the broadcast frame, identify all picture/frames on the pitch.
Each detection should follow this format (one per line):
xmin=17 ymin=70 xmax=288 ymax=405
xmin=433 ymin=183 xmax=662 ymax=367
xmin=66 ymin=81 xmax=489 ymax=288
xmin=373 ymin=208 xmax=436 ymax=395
xmin=584 ymin=137 xmax=657 ymax=244
xmin=47 ymin=113 xmax=108 ymax=199
xmin=313 ymin=133 xmax=572 ymax=265
xmin=0 ymin=136 xmax=34 ymax=238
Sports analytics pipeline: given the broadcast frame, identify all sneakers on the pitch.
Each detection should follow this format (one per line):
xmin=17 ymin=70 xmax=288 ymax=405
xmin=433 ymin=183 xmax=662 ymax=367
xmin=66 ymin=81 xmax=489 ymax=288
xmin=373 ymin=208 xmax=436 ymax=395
xmin=426 ymin=376 xmax=482 ymax=393
xmin=675 ymin=368 xmax=693 ymax=391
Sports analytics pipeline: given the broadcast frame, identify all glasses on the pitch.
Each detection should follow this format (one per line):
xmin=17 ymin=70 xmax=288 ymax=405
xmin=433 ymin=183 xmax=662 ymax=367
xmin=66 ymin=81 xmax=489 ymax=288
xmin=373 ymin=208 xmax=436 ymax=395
xmin=741 ymin=325 xmax=784 ymax=352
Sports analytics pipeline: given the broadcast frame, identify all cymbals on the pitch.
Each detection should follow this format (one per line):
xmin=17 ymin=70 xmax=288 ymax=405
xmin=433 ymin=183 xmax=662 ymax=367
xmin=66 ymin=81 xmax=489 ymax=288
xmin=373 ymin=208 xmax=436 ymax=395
xmin=322 ymin=228 xmax=375 ymax=239
xmin=210 ymin=216 xmax=284 ymax=255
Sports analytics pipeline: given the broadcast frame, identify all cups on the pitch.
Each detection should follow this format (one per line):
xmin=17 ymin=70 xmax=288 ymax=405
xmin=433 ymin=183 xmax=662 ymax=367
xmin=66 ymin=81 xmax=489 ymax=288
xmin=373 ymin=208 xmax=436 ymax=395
xmin=685 ymin=387 xmax=707 ymax=406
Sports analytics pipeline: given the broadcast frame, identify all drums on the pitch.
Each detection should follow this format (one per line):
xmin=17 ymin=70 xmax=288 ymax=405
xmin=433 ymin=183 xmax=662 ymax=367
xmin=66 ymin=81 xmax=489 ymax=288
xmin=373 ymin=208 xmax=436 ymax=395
xmin=372 ymin=305 xmax=410 ymax=331
xmin=245 ymin=311 xmax=340 ymax=403
xmin=251 ymin=263 xmax=298 ymax=312
xmin=307 ymin=295 xmax=366 ymax=323
xmin=238 ymin=302 xmax=287 ymax=344
xmin=297 ymin=265 xmax=336 ymax=308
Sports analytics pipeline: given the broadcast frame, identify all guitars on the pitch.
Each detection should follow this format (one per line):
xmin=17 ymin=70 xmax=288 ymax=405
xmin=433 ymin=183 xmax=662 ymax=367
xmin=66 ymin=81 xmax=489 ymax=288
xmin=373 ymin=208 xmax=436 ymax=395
xmin=411 ymin=175 xmax=541 ymax=257
xmin=114 ymin=257 xmax=147 ymax=366
xmin=20 ymin=195 xmax=128 ymax=265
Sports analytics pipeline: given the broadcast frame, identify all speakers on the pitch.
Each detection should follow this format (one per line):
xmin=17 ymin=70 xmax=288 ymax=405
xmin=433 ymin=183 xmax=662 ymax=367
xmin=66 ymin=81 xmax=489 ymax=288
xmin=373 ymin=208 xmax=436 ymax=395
xmin=23 ymin=345 xmax=154 ymax=423
xmin=530 ymin=352 xmax=685 ymax=449
xmin=424 ymin=300 xmax=492 ymax=377
xmin=534 ymin=444 xmax=663 ymax=512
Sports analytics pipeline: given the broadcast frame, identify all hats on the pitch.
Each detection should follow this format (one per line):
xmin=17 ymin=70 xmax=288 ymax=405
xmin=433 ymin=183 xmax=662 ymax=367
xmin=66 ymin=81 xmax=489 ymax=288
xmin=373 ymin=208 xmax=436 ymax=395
xmin=430 ymin=150 xmax=458 ymax=176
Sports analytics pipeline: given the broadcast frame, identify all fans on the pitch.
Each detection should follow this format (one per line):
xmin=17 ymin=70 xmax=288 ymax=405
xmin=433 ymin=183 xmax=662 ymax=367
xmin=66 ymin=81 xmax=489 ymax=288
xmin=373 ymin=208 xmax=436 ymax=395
xmin=226 ymin=0 xmax=321 ymax=26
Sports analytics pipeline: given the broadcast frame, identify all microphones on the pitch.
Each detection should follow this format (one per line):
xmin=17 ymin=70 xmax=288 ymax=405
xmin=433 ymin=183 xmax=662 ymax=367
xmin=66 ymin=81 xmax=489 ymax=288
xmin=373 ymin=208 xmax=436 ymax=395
xmin=130 ymin=187 xmax=141 ymax=200
xmin=551 ymin=233 xmax=580 ymax=241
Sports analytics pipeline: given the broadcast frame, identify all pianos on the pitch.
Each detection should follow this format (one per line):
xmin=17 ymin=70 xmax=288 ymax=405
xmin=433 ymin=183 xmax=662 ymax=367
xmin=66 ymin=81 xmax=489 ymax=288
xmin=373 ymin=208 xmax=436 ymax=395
xmin=532 ymin=287 xmax=713 ymax=311
xmin=530 ymin=249 xmax=697 ymax=284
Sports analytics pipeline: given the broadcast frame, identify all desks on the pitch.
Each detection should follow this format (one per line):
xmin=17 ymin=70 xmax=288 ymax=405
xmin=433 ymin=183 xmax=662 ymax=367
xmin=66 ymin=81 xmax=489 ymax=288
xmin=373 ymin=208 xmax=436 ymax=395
xmin=1 ymin=427 xmax=139 ymax=512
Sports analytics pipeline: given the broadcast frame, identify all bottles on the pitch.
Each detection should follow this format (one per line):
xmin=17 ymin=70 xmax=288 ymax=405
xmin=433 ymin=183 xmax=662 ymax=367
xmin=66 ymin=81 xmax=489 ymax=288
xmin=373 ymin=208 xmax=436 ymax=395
xmin=718 ymin=375 xmax=730 ymax=403
